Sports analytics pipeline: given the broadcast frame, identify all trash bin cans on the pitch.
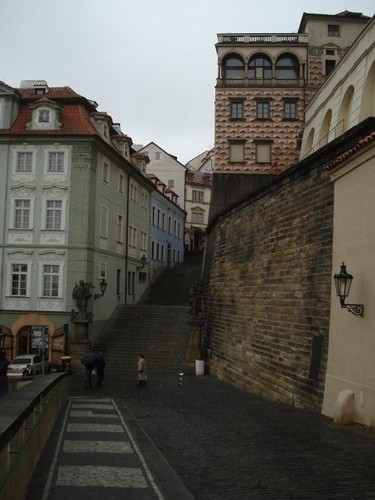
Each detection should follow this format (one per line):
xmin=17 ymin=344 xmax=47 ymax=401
xmin=61 ymin=355 xmax=72 ymax=374
xmin=195 ymin=359 xmax=205 ymax=376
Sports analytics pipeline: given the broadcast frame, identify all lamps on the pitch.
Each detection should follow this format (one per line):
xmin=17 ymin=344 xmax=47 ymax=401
xmin=136 ymin=254 xmax=147 ymax=271
xmin=334 ymin=262 xmax=363 ymax=318
xmin=94 ymin=279 xmax=108 ymax=300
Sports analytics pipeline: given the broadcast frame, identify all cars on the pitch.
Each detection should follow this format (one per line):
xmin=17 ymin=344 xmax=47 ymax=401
xmin=7 ymin=354 xmax=49 ymax=377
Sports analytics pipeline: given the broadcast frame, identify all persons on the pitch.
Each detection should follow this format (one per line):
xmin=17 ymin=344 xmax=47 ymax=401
xmin=137 ymin=354 xmax=146 ymax=386
xmin=81 ymin=350 xmax=106 ymax=389
xmin=75 ymin=281 xmax=91 ymax=316
xmin=23 ymin=365 xmax=33 ymax=376
